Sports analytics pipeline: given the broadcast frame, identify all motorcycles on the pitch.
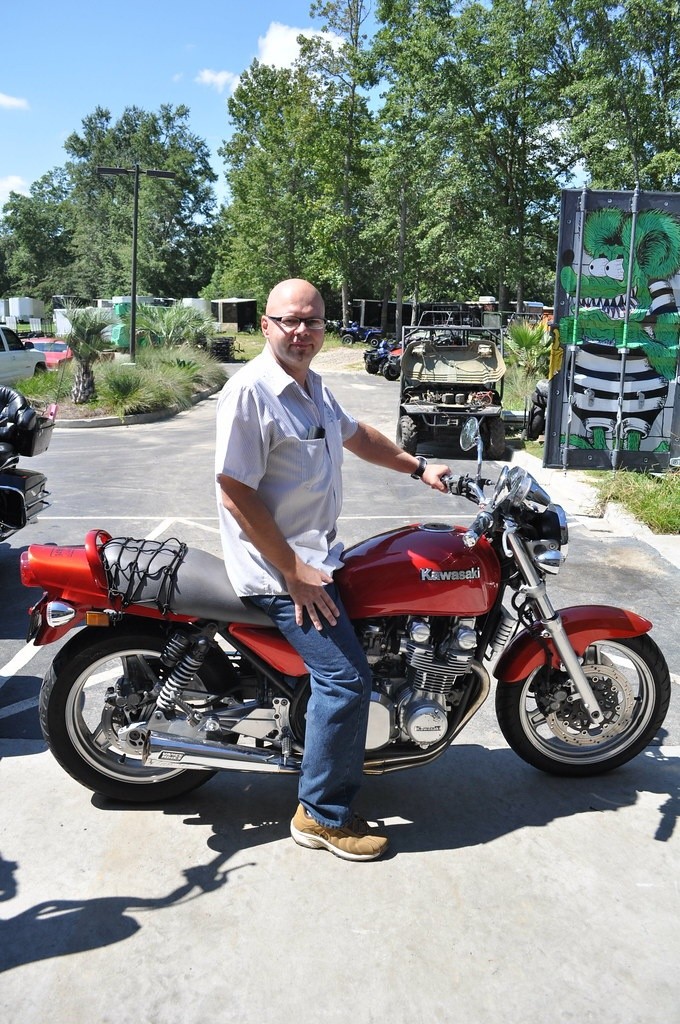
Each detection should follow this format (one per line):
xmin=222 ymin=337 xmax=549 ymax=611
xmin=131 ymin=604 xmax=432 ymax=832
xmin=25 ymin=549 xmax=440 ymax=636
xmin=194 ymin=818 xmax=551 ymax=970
xmin=362 ymin=337 xmax=404 ymax=381
xmin=343 ymin=323 xmax=382 ymax=347
xmin=23 ymin=420 xmax=675 ymax=783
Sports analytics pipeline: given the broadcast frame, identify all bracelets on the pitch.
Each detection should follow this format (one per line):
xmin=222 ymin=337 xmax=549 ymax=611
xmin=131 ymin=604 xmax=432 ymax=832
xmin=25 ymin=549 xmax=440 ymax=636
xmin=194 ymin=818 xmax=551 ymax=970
xmin=411 ymin=456 xmax=428 ymax=480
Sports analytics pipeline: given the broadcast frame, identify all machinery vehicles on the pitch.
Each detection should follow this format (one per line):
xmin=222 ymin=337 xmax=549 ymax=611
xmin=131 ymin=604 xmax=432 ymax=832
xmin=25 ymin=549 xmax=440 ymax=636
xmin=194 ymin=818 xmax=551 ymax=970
xmin=401 ymin=311 xmax=505 ymax=457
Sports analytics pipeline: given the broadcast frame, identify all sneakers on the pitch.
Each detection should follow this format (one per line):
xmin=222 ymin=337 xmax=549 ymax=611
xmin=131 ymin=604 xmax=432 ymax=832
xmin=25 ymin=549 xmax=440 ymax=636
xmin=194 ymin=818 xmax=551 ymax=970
xmin=289 ymin=803 xmax=387 ymax=862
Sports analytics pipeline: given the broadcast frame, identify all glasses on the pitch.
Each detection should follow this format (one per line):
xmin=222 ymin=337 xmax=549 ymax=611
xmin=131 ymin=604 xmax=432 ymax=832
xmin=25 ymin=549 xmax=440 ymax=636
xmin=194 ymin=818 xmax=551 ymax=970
xmin=266 ymin=315 xmax=327 ymax=330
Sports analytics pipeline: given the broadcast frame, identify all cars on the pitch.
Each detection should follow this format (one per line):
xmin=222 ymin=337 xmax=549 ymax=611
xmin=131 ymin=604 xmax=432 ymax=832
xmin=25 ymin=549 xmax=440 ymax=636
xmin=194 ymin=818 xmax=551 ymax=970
xmin=0 ymin=329 xmax=45 ymax=377
xmin=26 ymin=333 xmax=73 ymax=369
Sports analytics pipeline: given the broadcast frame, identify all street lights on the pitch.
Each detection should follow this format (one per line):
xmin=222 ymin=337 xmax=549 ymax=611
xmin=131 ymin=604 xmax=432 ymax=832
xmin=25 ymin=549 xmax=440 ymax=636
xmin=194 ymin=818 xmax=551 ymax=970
xmin=95 ymin=163 xmax=174 ymax=365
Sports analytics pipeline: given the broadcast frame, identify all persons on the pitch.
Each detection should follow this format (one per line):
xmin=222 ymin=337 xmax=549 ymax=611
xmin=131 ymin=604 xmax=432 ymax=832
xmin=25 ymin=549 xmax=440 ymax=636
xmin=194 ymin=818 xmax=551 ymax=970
xmin=216 ymin=278 xmax=450 ymax=862
